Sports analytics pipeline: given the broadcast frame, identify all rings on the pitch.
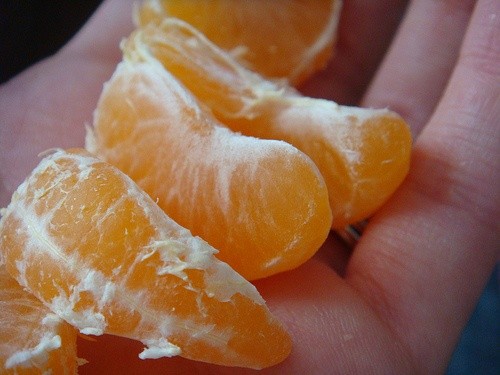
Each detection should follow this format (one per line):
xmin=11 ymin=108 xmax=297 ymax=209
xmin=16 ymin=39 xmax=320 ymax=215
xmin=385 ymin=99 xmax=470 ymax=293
xmin=332 ymin=224 xmax=362 ymax=250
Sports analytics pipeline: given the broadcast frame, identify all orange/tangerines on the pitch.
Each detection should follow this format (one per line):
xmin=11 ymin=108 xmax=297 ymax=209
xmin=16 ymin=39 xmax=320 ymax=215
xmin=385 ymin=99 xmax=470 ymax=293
xmin=0 ymin=0 xmax=412 ymax=375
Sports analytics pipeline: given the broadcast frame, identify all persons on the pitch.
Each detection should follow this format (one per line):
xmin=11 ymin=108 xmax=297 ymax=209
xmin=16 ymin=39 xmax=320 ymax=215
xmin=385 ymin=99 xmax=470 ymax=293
xmin=0 ymin=1 xmax=500 ymax=375
